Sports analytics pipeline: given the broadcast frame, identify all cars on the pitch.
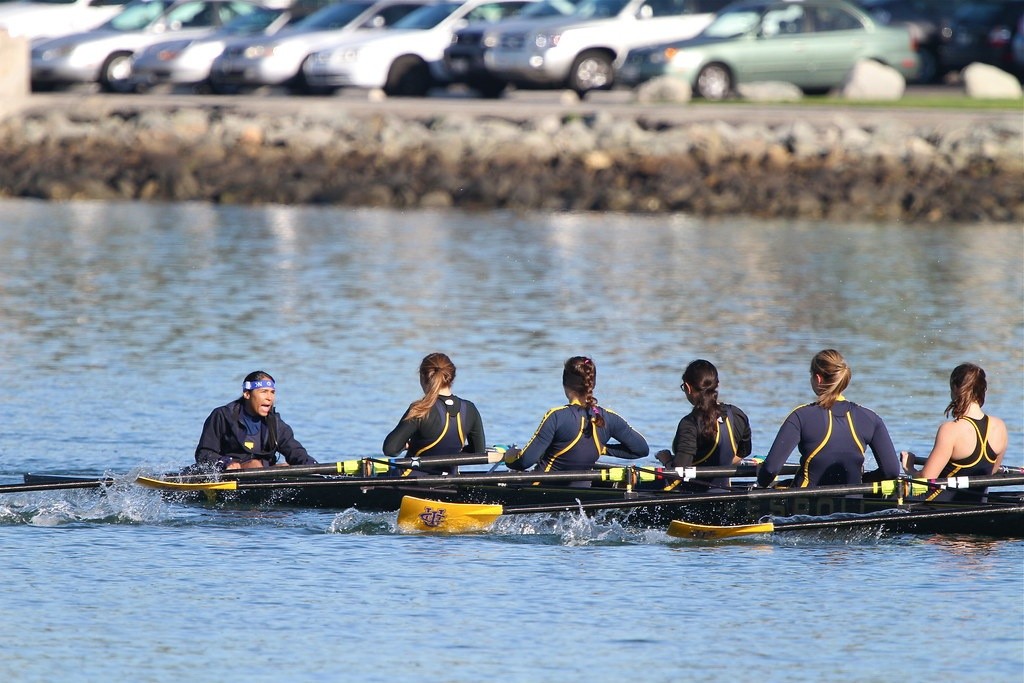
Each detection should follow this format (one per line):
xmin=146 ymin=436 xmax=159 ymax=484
xmin=619 ymin=1 xmax=925 ymax=103
xmin=475 ymin=1 xmax=757 ymax=94
xmin=27 ymin=0 xmax=280 ymax=96
xmin=211 ymin=1 xmax=381 ymax=99
xmin=0 ymin=1 xmax=139 ymax=45
xmin=299 ymin=0 xmax=500 ymax=98
xmin=866 ymin=0 xmax=1024 ymax=87
xmin=440 ymin=1 xmax=577 ymax=103
xmin=130 ymin=3 xmax=310 ymax=99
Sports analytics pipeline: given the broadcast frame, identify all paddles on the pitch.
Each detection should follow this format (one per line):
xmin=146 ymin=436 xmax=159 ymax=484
xmin=133 ymin=464 xmax=771 ymax=493
xmin=675 ymin=503 xmax=1023 ymax=541
xmin=396 ymin=477 xmax=935 ymax=533
xmin=900 ymin=451 xmax=1024 ymax=476
xmin=0 ymin=457 xmax=401 ymax=493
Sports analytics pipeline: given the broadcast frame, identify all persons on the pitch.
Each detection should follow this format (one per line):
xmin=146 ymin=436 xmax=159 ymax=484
xmin=892 ymin=363 xmax=1007 ymax=503
xmin=654 ymin=359 xmax=752 ymax=493
xmin=754 ymin=349 xmax=900 ymax=497
xmin=504 ymin=356 xmax=650 ymax=488
xmin=382 ymin=353 xmax=486 ymax=476
xmin=195 ymin=371 xmax=320 ymax=475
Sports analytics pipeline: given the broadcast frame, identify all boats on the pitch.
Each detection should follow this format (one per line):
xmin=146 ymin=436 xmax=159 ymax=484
xmin=22 ymin=466 xmax=1023 ymax=539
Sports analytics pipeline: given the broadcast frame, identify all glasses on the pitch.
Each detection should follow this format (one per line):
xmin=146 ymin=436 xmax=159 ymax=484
xmin=680 ymin=382 xmax=686 ymax=391
